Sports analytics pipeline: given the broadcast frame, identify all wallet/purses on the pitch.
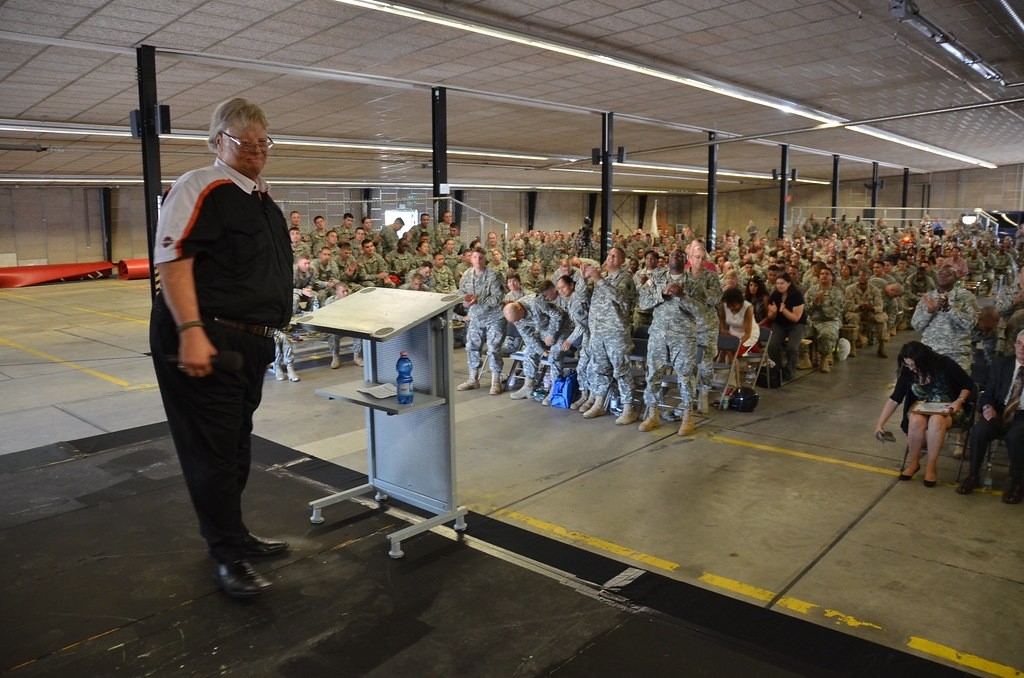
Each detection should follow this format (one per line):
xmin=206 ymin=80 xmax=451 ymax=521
xmin=877 ymin=431 xmax=896 ymax=442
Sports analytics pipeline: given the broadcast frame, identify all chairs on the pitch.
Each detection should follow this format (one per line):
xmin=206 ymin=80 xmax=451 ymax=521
xmin=900 ymin=382 xmax=1012 ymax=483
xmin=479 ymin=321 xmax=772 ymax=421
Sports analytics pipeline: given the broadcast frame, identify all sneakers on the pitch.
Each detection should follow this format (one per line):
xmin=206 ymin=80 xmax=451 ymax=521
xmin=1003 ymin=483 xmax=1024 ymax=504
xmin=958 ymin=476 xmax=978 ymax=494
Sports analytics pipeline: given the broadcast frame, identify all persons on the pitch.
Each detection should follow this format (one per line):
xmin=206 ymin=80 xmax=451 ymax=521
xmin=461 ymin=213 xmax=1024 ymax=504
xmin=149 ymin=98 xmax=295 ymax=597
xmin=274 ymin=211 xmax=474 ymax=381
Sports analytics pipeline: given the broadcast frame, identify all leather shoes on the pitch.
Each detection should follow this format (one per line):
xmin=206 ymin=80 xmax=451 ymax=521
xmin=214 ymin=558 xmax=273 ymax=596
xmin=243 ymin=533 xmax=288 ymax=557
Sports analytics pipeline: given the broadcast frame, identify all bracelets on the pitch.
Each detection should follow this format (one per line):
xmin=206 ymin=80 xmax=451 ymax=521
xmin=957 ymin=397 xmax=965 ymax=402
xmin=982 ymin=405 xmax=989 ymax=410
xmin=177 ymin=321 xmax=204 ymax=332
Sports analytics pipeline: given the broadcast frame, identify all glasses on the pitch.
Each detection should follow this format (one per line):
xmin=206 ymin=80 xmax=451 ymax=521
xmin=222 ymin=132 xmax=274 ymax=150
xmin=904 ymin=364 xmax=916 ymax=368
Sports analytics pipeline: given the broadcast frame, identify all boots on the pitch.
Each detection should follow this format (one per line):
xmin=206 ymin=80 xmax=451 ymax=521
xmin=457 ymin=369 xmax=480 ymax=390
xmin=287 ymin=363 xmax=300 ymax=382
xmin=490 ymin=377 xmax=708 ymax=436
xmin=782 ymin=320 xmax=909 ymax=372
xmin=331 ymin=352 xmax=339 ymax=368
xmin=985 ymin=291 xmax=993 ymax=297
xmin=275 ymin=365 xmax=285 ymax=381
xmin=307 ymin=330 xmax=320 ymax=337
xmin=292 ymin=325 xmax=303 ymax=341
xmin=354 ymin=352 xmax=364 ymax=367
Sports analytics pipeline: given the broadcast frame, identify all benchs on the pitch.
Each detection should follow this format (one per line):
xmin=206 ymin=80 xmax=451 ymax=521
xmin=268 ymin=331 xmax=356 ymax=374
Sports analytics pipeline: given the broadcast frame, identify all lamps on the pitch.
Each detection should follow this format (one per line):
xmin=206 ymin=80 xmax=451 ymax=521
xmin=0 ymin=0 xmax=997 ymax=196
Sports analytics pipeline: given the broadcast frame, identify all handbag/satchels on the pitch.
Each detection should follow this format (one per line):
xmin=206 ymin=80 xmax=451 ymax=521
xmin=755 ymin=365 xmax=782 ymax=388
xmin=551 ymin=372 xmax=578 ymax=409
xmin=912 ymin=401 xmax=952 ymax=415
xmin=728 ymin=387 xmax=760 ymax=412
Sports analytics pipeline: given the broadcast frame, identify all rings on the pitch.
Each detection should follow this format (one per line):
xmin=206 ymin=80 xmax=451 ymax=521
xmin=178 ymin=365 xmax=185 ymax=368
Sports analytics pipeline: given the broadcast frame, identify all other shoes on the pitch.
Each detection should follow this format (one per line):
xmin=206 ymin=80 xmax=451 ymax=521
xmin=924 ymin=480 xmax=936 ymax=487
xmin=899 ymin=465 xmax=920 ymax=481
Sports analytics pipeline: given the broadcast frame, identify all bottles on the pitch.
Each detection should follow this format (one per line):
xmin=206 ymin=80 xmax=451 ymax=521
xmin=722 ymin=394 xmax=729 ymax=409
xmin=815 ymin=352 xmax=821 ymax=365
xmin=610 ymin=392 xmax=618 ymax=411
xmin=508 ymin=370 xmax=516 ymax=385
xmin=746 ymin=365 xmax=756 ymax=387
xmin=313 ymin=299 xmax=319 ymax=311
xmin=396 ymin=352 xmax=413 ymax=404
xmin=983 ymin=464 xmax=992 ymax=492
xmin=543 ymin=374 xmax=550 ymax=388
xmin=702 ymin=385 xmax=709 ymax=413
xmin=872 ymin=338 xmax=876 ymax=344
xmin=294 ymin=331 xmax=299 ymax=339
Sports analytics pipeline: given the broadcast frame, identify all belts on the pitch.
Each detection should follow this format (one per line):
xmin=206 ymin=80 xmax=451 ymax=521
xmin=212 ymin=316 xmax=275 ymax=338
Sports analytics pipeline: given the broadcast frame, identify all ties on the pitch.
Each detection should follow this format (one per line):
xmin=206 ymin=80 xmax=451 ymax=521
xmin=1003 ymin=366 xmax=1024 ymax=423
